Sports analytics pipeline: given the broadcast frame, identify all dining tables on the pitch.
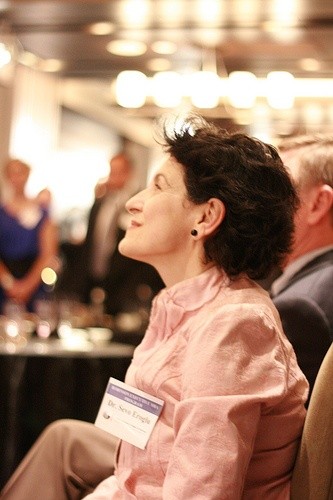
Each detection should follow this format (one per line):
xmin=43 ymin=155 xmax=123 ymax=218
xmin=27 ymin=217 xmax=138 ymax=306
xmin=0 ymin=316 xmax=135 ymax=480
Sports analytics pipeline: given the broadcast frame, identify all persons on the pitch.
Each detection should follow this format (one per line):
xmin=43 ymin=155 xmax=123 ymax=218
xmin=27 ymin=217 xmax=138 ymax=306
xmin=0 ymin=160 xmax=60 ymax=317
xmin=0 ymin=133 xmax=332 ymax=500
xmin=82 ymin=154 xmax=165 ymax=314
xmin=78 ymin=126 xmax=310 ymax=499
